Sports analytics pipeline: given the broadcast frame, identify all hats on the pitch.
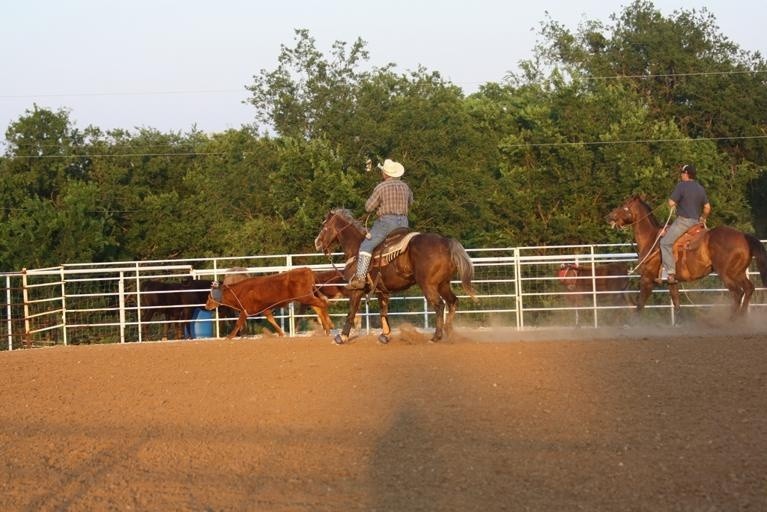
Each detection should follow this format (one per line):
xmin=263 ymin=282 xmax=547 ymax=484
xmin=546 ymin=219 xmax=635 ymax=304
xmin=377 ymin=159 xmax=405 ymax=177
xmin=681 ymin=164 xmax=696 ymax=175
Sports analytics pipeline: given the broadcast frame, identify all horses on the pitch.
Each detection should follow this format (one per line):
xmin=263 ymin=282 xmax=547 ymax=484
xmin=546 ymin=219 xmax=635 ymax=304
xmin=607 ymin=193 xmax=767 ymax=329
xmin=314 ymin=208 xmax=480 ymax=346
xmin=294 ymin=270 xmax=362 ymax=335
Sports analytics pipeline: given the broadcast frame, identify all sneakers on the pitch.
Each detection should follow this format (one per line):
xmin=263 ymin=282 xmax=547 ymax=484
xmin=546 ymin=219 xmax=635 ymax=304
xmin=654 ymin=275 xmax=676 ymax=285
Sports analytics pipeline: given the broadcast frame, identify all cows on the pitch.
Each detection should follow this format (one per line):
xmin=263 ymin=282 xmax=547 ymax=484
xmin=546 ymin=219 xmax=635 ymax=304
xmin=136 ymin=266 xmax=249 ymax=341
xmin=204 ymin=267 xmax=337 ymax=340
xmin=558 ymin=263 xmax=637 ymax=330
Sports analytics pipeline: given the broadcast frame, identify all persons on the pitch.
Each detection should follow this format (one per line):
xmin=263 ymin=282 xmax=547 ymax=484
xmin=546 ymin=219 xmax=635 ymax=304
xmin=346 ymin=159 xmax=413 ymax=289
xmin=655 ymin=165 xmax=711 ymax=284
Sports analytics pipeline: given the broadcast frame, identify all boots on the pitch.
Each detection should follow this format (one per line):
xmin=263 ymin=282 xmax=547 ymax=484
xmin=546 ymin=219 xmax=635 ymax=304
xmin=345 ymin=251 xmax=372 ymax=289
